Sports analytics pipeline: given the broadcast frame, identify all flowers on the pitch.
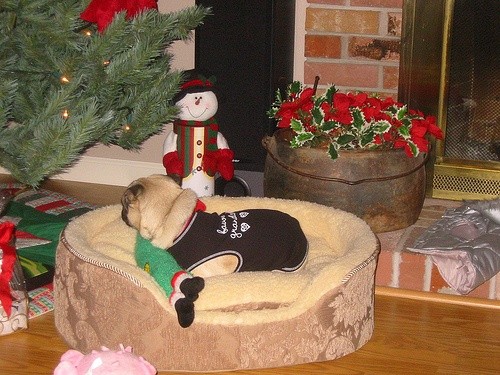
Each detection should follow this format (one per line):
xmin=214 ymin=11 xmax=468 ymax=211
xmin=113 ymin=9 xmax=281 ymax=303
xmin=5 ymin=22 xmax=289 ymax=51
xmin=265 ymin=81 xmax=445 ymax=160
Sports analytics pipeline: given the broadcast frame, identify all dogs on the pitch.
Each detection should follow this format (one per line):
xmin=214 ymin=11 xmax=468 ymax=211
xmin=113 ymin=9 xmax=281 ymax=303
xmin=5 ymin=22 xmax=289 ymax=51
xmin=121 ymin=173 xmax=311 ymax=279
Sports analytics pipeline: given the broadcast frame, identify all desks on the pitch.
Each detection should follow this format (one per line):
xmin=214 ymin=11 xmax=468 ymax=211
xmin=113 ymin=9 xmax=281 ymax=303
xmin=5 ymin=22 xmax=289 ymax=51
xmin=1 ymin=173 xmax=499 ymax=375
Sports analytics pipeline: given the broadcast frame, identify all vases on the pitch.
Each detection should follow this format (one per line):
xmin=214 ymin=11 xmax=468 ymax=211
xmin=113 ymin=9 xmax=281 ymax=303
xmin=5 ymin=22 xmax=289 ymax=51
xmin=260 ymin=128 xmax=433 ymax=235
